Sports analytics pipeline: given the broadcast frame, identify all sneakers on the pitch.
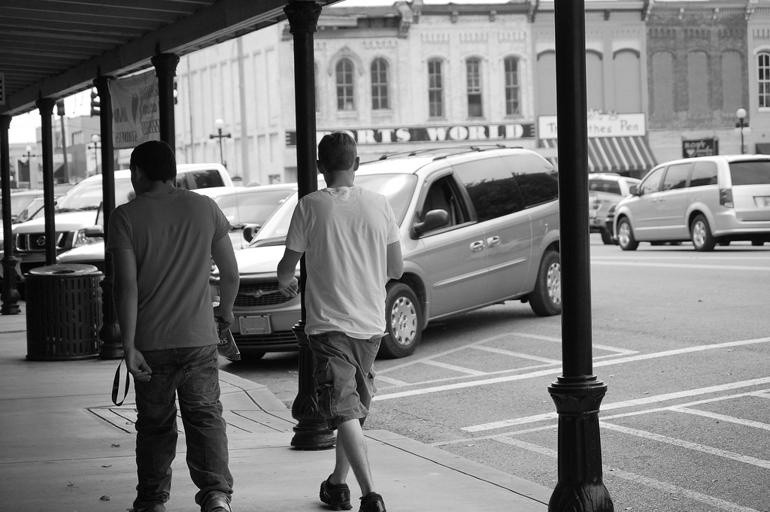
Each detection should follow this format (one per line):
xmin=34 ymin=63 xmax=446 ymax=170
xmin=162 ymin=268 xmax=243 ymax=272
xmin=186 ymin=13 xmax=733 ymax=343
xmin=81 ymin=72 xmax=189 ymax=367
xmin=358 ymin=492 xmax=386 ymax=512
xmin=200 ymin=491 xmax=231 ymax=512
xmin=319 ymin=474 xmax=353 ymax=510
xmin=127 ymin=503 xmax=166 ymax=512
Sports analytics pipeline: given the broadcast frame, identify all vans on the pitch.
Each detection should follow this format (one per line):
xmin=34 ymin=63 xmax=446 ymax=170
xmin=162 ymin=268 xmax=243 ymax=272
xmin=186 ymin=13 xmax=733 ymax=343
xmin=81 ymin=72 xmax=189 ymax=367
xmin=606 ymin=153 xmax=770 ymax=250
xmin=209 ymin=143 xmax=562 ymax=359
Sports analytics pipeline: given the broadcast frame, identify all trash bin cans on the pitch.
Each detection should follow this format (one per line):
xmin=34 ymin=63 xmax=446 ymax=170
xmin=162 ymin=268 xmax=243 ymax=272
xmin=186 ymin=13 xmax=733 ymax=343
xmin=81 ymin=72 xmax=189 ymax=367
xmin=24 ymin=263 xmax=104 ymax=361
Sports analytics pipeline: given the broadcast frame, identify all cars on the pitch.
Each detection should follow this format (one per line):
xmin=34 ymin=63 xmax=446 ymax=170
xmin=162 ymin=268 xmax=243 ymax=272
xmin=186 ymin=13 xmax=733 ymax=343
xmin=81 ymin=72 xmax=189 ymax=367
xmin=588 ymin=172 xmax=641 ymax=245
xmin=55 ymin=183 xmax=299 ymax=269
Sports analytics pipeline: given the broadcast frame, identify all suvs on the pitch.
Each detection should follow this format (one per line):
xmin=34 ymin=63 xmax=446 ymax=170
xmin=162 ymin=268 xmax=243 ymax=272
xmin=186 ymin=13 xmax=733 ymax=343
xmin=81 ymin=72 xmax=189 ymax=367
xmin=0 ymin=163 xmax=235 ymax=287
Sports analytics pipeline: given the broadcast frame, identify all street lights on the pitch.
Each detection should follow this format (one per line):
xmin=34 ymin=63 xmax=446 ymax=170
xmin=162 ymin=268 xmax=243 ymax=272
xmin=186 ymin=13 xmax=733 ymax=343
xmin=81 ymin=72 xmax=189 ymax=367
xmin=736 ymin=108 xmax=747 ymax=153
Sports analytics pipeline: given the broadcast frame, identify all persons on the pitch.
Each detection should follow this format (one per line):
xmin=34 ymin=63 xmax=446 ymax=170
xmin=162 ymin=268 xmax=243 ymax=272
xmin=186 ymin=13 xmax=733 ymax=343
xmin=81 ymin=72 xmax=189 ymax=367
xmin=103 ymin=139 xmax=245 ymax=512
xmin=273 ymin=131 xmax=406 ymax=512
